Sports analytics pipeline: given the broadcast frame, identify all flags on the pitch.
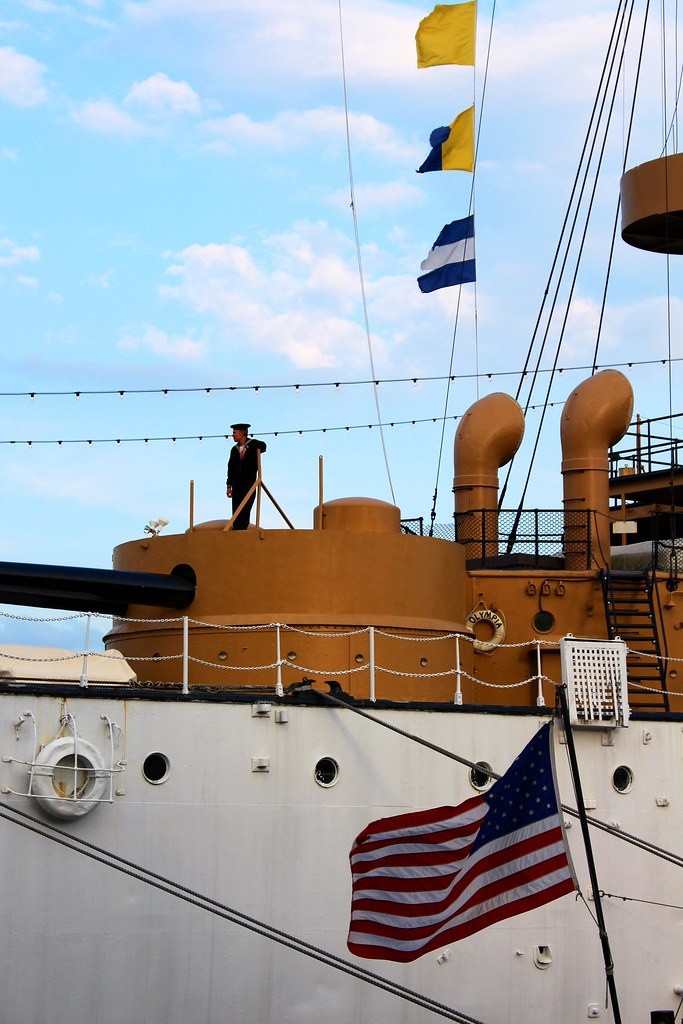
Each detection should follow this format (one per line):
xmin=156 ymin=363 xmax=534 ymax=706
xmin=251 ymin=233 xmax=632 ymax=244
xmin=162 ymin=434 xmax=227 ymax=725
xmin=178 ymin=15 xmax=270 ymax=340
xmin=416 ymin=214 xmax=476 ymax=294
xmin=414 ymin=105 xmax=475 ymax=173
xmin=346 ymin=722 xmax=580 ymax=964
xmin=415 ymin=1 xmax=477 ymax=68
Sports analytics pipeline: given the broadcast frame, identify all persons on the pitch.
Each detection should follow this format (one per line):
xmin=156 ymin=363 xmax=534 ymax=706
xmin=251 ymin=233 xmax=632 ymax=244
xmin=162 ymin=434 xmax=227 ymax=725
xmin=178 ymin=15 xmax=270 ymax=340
xmin=225 ymin=424 xmax=267 ymax=530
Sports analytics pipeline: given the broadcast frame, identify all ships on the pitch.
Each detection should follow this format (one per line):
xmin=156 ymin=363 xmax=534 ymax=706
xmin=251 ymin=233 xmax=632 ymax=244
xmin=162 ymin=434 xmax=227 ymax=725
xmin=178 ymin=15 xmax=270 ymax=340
xmin=0 ymin=2 xmax=682 ymax=1024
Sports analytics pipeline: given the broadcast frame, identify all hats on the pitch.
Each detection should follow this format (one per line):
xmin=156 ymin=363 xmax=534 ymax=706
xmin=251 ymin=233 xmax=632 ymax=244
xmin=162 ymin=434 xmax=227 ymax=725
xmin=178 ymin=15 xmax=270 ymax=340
xmin=230 ymin=424 xmax=251 ymax=430
